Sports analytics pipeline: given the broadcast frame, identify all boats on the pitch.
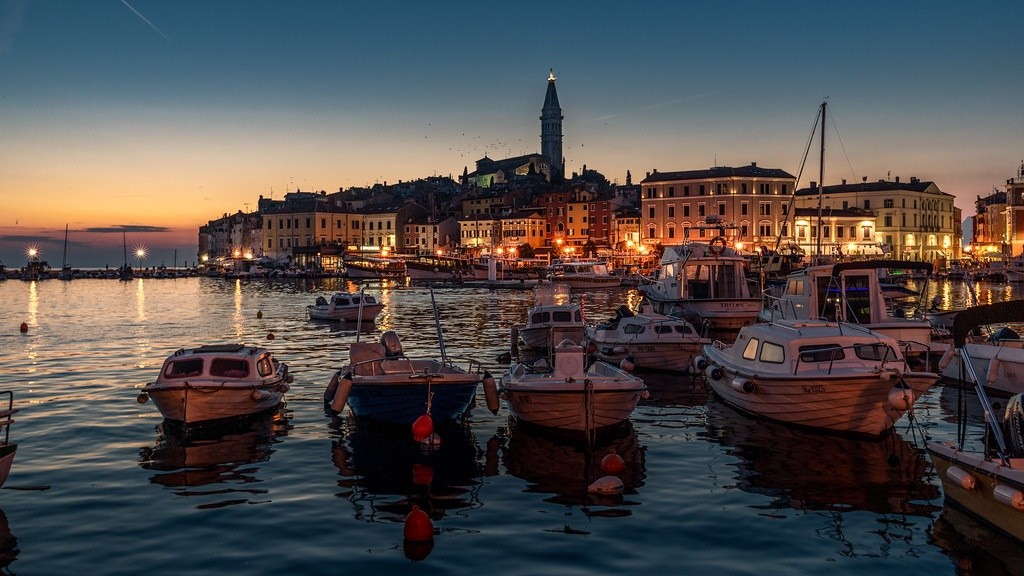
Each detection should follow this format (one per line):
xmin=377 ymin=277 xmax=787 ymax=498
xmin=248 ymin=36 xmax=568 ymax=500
xmin=519 ymin=305 xmax=587 ymax=349
xmin=137 ymin=345 xmax=294 ymax=423
xmin=343 ymin=257 xmax=409 ymax=279
xmin=759 ymin=261 xmax=936 ymax=361
xmin=306 ymin=292 xmax=386 ymax=321
xmin=949 ymin=268 xmax=1024 ymax=283
xmin=703 ymin=320 xmax=940 ymax=436
xmin=636 ymin=229 xmax=767 ymax=328
xmin=324 ymin=287 xmax=500 ymax=446
xmin=917 ymin=295 xmax=982 ymax=331
xmin=546 ymin=260 xmax=621 ymax=287
xmin=927 ymin=392 xmax=1024 ymax=541
xmin=587 ymin=304 xmax=712 ymax=373
xmin=495 ymin=321 xmax=650 ymax=432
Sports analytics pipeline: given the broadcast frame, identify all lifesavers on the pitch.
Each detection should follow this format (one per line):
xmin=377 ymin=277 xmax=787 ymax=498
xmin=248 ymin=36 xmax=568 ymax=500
xmin=708 ymin=236 xmax=726 ymax=256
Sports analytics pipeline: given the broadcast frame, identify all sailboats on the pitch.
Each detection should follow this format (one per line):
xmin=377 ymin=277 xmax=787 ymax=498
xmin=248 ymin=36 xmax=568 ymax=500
xmin=745 ymin=96 xmax=925 ymax=318
